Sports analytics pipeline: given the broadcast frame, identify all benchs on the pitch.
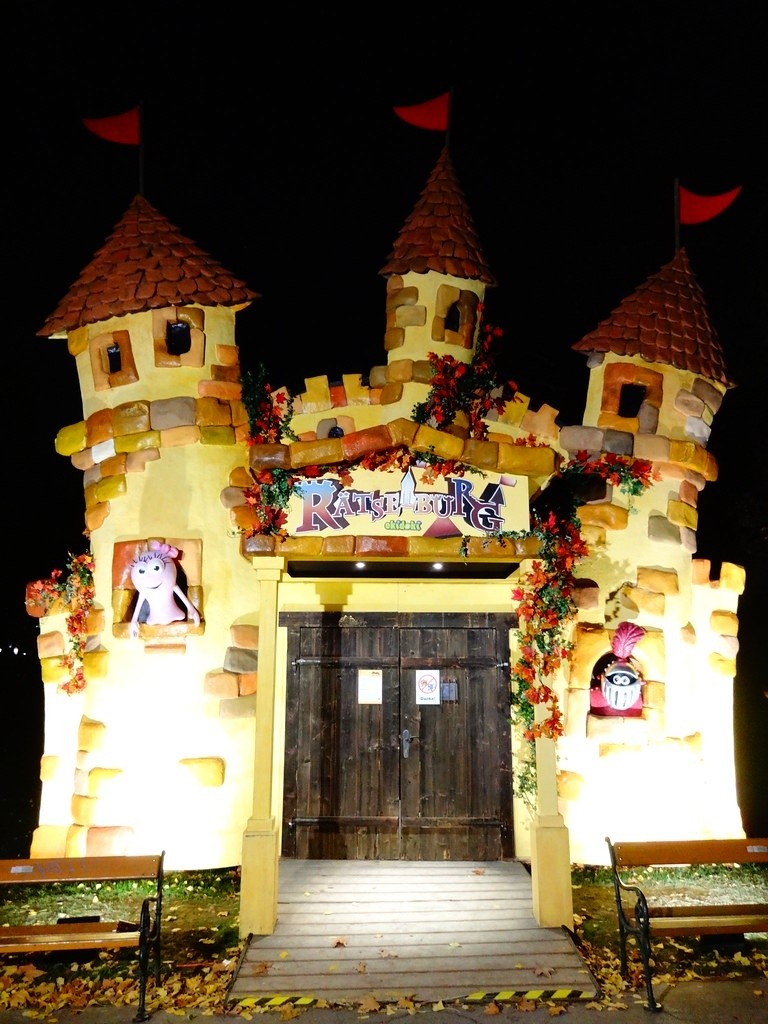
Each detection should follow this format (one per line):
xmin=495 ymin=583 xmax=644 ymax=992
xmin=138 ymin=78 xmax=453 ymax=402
xmin=0 ymin=850 xmax=166 ymax=1023
xmin=605 ymin=836 xmax=768 ymax=1012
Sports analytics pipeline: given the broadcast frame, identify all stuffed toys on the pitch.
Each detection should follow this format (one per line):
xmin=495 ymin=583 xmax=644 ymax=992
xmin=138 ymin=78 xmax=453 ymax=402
xmin=129 ymin=540 xmax=200 ymax=638
